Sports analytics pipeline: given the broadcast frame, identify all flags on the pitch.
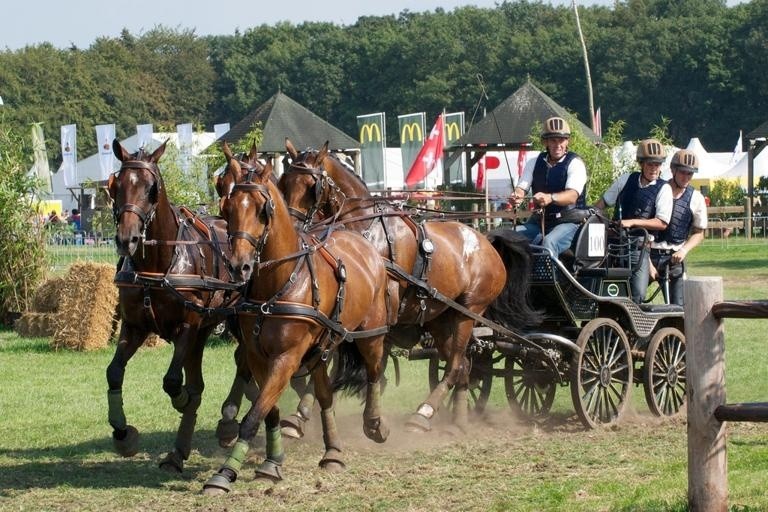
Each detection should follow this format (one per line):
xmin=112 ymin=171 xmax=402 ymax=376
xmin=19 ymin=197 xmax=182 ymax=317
xmin=477 ymin=143 xmax=484 ymax=193
xmin=403 ymin=112 xmax=443 ymax=189
xmin=516 ymin=141 xmax=526 ymax=184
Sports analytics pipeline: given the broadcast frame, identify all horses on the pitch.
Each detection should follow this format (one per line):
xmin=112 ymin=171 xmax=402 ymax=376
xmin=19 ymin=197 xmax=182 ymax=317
xmin=105 ymin=134 xmax=552 ymax=498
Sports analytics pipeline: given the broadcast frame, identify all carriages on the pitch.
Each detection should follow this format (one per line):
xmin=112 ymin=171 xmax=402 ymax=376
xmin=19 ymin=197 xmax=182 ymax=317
xmin=104 ymin=139 xmax=687 ymax=497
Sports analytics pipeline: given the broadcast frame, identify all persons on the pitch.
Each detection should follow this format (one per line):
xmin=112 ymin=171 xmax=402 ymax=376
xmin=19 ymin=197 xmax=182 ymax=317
xmin=648 ymin=149 xmax=709 ymax=308
xmin=508 ymin=116 xmax=589 ymax=281
xmin=33 ymin=208 xmax=80 ymax=225
xmin=592 ymin=139 xmax=673 ymax=301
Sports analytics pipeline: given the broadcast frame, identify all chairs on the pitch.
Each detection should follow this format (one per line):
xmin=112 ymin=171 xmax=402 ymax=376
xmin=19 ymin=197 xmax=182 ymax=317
xmin=490 ymin=208 xmax=648 ymax=323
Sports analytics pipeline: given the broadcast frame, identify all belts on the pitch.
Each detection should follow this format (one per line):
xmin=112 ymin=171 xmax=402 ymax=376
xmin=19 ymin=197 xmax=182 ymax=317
xmin=653 ymin=249 xmax=676 ymax=254
xmin=537 ymin=212 xmax=561 ymax=221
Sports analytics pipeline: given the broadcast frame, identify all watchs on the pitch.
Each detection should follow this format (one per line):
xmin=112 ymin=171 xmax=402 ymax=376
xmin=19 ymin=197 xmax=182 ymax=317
xmin=550 ymin=192 xmax=558 ymax=204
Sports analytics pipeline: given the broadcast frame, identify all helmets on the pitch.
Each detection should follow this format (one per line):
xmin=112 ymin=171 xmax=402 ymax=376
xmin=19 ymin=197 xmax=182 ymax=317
xmin=637 ymin=139 xmax=667 ymax=163
xmin=541 ymin=117 xmax=571 ymax=139
xmin=672 ymin=150 xmax=699 ymax=173
